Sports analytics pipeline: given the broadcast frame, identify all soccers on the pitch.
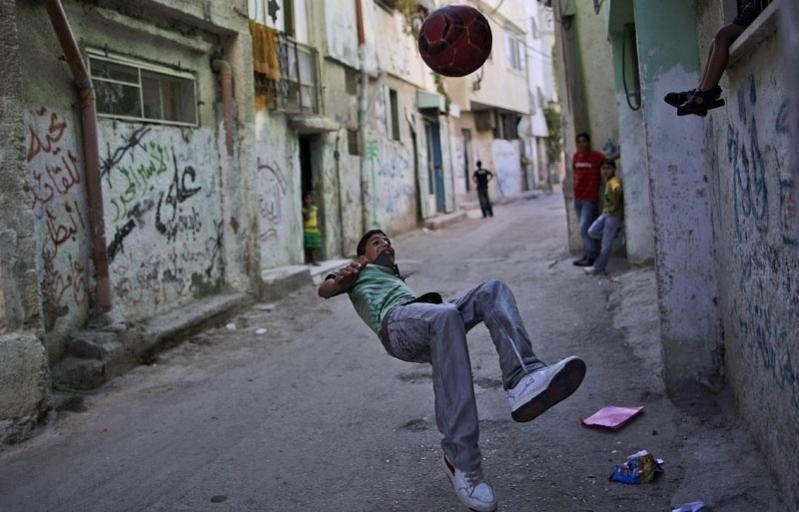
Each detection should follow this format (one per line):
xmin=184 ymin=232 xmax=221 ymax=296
xmin=418 ymin=4 xmax=492 ymax=77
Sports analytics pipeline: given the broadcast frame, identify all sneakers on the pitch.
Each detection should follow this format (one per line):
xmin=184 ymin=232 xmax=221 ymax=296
xmin=440 ymin=450 xmax=498 ymax=511
xmin=572 ymin=257 xmax=605 ymax=274
xmin=505 ymin=355 xmax=588 ymax=423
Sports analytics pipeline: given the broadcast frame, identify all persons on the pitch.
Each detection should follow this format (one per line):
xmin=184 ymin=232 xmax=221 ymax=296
xmin=664 ymin=2 xmax=761 ymax=116
xmin=583 ymin=159 xmax=623 ymax=275
xmin=302 ymin=192 xmax=323 ymax=265
xmin=319 ymin=228 xmax=586 ymax=511
xmin=471 ymin=160 xmax=493 ymax=217
xmin=573 ymin=134 xmax=623 ymax=266
